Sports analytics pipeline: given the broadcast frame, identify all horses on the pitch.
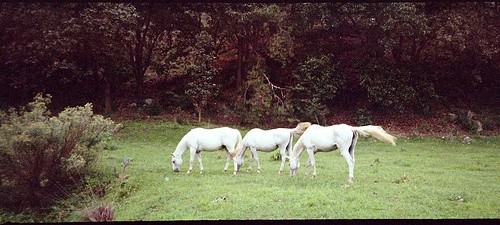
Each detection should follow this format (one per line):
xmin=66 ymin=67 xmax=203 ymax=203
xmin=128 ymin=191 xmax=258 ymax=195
xmin=235 ymin=122 xmax=310 ymax=174
xmin=289 ymin=123 xmax=397 ymax=186
xmin=171 ymin=126 xmax=241 ymax=174
